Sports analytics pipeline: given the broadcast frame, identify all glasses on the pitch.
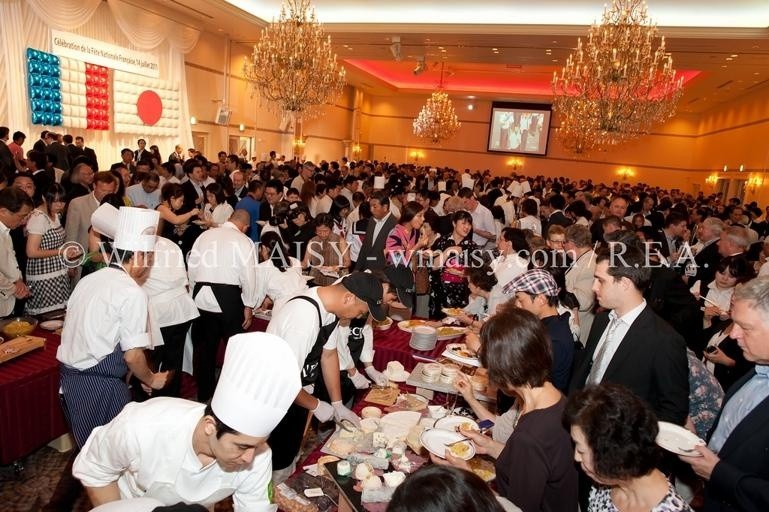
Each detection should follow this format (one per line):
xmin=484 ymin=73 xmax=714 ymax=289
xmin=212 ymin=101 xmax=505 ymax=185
xmin=415 ymin=199 xmax=426 ymax=201
xmin=264 ymin=192 xmax=278 ymax=196
xmin=549 ymin=239 xmax=564 ymax=243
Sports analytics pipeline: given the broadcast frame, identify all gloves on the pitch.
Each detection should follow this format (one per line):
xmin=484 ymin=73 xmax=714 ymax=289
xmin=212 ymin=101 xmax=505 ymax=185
xmin=346 ymin=369 xmax=371 ymax=390
xmin=331 ymin=400 xmax=361 ymax=431
xmin=365 ymin=366 xmax=388 ymax=388
xmin=312 ymin=399 xmax=334 ymax=423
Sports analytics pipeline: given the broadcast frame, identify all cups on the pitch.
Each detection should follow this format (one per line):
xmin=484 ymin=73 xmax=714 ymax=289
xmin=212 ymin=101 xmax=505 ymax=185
xmin=471 ymin=376 xmax=487 ymax=392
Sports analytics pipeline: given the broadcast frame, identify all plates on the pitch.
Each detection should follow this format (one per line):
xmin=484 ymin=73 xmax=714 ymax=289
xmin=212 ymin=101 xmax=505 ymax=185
xmin=420 ymin=363 xmax=441 ymax=383
xmin=447 ymin=343 xmax=483 ymax=359
xmin=40 ymin=319 xmax=65 ymax=331
xmin=398 ymin=320 xmax=432 ymax=333
xmin=382 ymin=370 xmax=410 ymax=383
xmin=409 ymin=325 xmax=438 ymax=351
xmin=441 ymin=364 xmax=458 ymax=384
xmin=191 ymin=219 xmax=207 ymax=226
xmin=397 ymin=393 xmax=427 ymax=411
xmin=436 ymin=327 xmax=468 ymax=341
xmin=433 ymin=414 xmax=480 ymax=433
xmin=372 ymin=315 xmax=392 ymax=331
xmin=654 ymin=420 xmax=709 ymax=457
xmin=441 ymin=307 xmax=471 ymax=317
xmin=419 ymin=428 xmax=477 ymax=462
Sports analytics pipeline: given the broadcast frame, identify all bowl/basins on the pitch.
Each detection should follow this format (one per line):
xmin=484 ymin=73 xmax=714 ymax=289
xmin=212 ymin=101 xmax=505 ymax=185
xmin=1 ymin=315 xmax=38 ymax=338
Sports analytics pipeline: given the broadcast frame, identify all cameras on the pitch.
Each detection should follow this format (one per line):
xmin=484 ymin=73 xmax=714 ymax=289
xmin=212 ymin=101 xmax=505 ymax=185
xmin=269 ymin=201 xmax=300 ymax=226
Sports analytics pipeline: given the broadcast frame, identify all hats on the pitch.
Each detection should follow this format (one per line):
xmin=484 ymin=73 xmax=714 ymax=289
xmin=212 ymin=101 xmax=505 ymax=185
xmin=462 ymin=172 xmax=474 ymax=190
xmin=506 ymin=180 xmax=520 ymax=193
xmin=384 ymin=264 xmax=416 ymax=308
xmin=374 ymin=176 xmax=384 ymax=189
xmin=260 ymin=153 xmax=267 ymax=161
xmin=521 ymin=181 xmax=531 ymax=193
xmin=342 ymin=271 xmax=387 ymax=322
xmin=90 ymin=202 xmax=118 ymax=239
xmin=438 ymin=182 xmax=446 ymax=192
xmin=510 ymin=186 xmax=522 ymax=198
xmin=251 ymin=151 xmax=257 ymax=157
xmin=502 ymin=269 xmax=560 ymax=297
xmin=211 ymin=332 xmax=304 ymax=438
xmin=114 ymin=206 xmax=161 ymax=250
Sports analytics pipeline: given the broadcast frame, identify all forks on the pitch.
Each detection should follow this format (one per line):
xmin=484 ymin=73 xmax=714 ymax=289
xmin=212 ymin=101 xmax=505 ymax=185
xmin=196 ymin=206 xmax=198 ymax=209
xmin=676 ymin=447 xmax=707 ymax=453
xmin=443 ymin=437 xmax=472 ymax=447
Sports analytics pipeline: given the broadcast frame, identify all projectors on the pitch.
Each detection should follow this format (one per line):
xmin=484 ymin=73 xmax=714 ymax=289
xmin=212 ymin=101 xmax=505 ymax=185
xmin=413 ymin=56 xmax=424 ymax=74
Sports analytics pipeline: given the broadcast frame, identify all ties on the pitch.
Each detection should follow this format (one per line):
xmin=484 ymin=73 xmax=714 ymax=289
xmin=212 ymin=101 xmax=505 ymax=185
xmin=586 ymin=318 xmax=621 ymax=386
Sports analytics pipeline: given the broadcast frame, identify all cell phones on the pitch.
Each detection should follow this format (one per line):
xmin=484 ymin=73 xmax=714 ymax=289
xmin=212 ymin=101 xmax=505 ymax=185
xmin=707 ymin=346 xmax=715 ymax=352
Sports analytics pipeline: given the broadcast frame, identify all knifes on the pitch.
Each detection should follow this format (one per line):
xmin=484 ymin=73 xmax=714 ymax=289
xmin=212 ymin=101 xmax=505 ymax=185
xmin=332 ymin=416 xmax=354 ymax=433
xmin=370 ymin=384 xmax=390 ymax=389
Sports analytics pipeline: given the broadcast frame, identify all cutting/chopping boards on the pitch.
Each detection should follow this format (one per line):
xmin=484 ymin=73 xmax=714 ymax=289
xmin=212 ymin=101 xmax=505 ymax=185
xmin=1 ymin=335 xmax=46 ymax=363
xmin=363 ymin=384 xmax=401 ymax=406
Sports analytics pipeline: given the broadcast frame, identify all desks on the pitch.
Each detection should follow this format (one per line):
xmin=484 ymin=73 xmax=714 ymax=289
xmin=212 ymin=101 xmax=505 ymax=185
xmin=0 ymin=312 xmax=496 ymax=512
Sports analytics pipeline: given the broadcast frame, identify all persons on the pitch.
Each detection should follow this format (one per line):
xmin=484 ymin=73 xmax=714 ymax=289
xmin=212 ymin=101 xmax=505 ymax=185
xmin=589 ymin=206 xmax=602 ymax=221
xmin=261 ymin=272 xmax=386 ymax=487
xmin=429 ymin=210 xmax=478 ymax=322
xmin=91 ymin=202 xmax=200 ymax=399
xmin=208 ymin=148 xmax=302 ymax=182
xmin=458 ymin=187 xmax=497 ymax=248
xmin=512 ymin=198 xmax=542 ymax=236
xmin=160 ymin=162 xmax=182 ymax=184
xmin=299 ymin=182 xmax=316 ymax=218
xmin=33 ymin=131 xmax=50 ymax=151
xmin=184 ymin=147 xmax=208 ymax=166
xmin=700 ymin=225 xmax=757 ymax=283
xmin=603 ymin=216 xmax=622 ymax=233
xmin=493 ymin=181 xmax=519 ymax=206
xmin=135 ymin=139 xmax=152 ymax=160
xmin=315 ymin=156 xmax=359 ymax=178
xmin=199 ymin=183 xmax=234 ymax=229
xmin=386 ymin=201 xmax=431 ymax=267
xmin=0 ymin=186 xmax=33 ymax=319
xmin=188 ymin=209 xmax=256 ymax=404
xmin=429 ymin=307 xmax=580 ymax=512
xmin=359 ymin=159 xmax=495 ymax=201
xmin=382 ymin=466 xmax=506 ymax=511
xmin=589 ymin=182 xmax=612 ymax=210
xmin=328 ymin=195 xmax=350 ymax=237
xmin=757 ymin=236 xmax=769 ymax=276
xmin=27 ymin=150 xmax=55 ymax=208
xmin=258 ymin=178 xmax=290 ymax=221
xmin=689 ymin=259 xmax=742 ymax=323
xmin=13 ymin=173 xmax=35 ymax=198
xmin=529 ymin=236 xmax=545 ymax=250
xmin=44 ymin=132 xmax=68 ymax=171
xmin=567 ymin=244 xmax=691 ymax=432
xmin=542 ymin=175 xmax=592 ymax=202
xmin=688 ymin=190 xmax=769 ymax=238
xmin=9 ymin=131 xmax=26 ymax=172
xmin=291 ymin=161 xmax=316 ymax=193
xmin=317 ymin=263 xmax=414 ymax=445
xmin=456 ymin=264 xmax=508 ymax=335
xmin=353 ymin=192 xmax=398 ymax=282
xmin=155 ymin=183 xmax=199 ymax=236
xmin=677 ymin=277 xmax=769 ymax=511
xmin=233 ymin=172 xmax=247 ymax=203
xmin=565 ymin=204 xmax=570 ymax=217
xmin=61 ymin=163 xmax=94 ymax=228
xmin=114 ymin=168 xmax=130 ymax=186
xmin=125 ymin=171 xmax=162 ymax=209
xmin=496 ymin=172 xmax=545 ymax=181
xmin=256 ymin=231 xmax=308 ymax=315
xmin=547 ymin=195 xmax=572 ymax=227
xmin=80 ymin=194 xmax=126 ymax=278
xmin=56 ymin=206 xmax=169 ymax=451
xmin=286 ymin=188 xmax=299 ymax=203
xmin=654 ymin=185 xmax=688 ymax=212
xmin=629 ymin=182 xmax=654 ymax=213
xmin=136 ymin=160 xmax=155 ymax=177
xmin=653 ymin=214 xmax=688 ymax=319
xmin=632 ymin=214 xmax=645 ymax=229
xmin=75 ymin=136 xmax=98 ymax=172
xmin=517 ymin=181 xmax=541 ymax=217
xmin=345 ymin=190 xmax=366 ymax=272
xmin=278 ymin=201 xmax=315 ymax=261
xmin=502 ymin=269 xmax=576 ymax=397
xmin=612 ymin=181 xmax=632 ymax=196
xmin=111 ymin=171 xmax=131 ymax=207
xmin=564 ymin=223 xmax=600 ymax=348
xmin=300 ymin=212 xmax=352 ymax=286
xmin=416 ymin=190 xmax=439 ymax=224
xmin=562 ymin=384 xmax=693 ymax=511
xmin=26 ymin=182 xmax=83 ymax=319
xmin=680 ymin=218 xmax=729 ymax=305
xmin=546 ymin=224 xmax=566 ymax=249
xmin=317 ymin=178 xmax=342 ymax=213
xmin=502 ymin=185 xmax=522 ymax=225
xmin=182 ymin=163 xmax=207 ymax=209
xmin=64 ymin=171 xmax=116 ymax=291
xmin=151 ymin=145 xmax=161 ymax=165
xmin=609 ymin=197 xmax=629 ymax=219
xmin=490 ymin=227 xmax=531 ymax=287
xmin=447 ymin=197 xmax=464 ymax=213
xmin=234 ymin=180 xmax=264 ymax=242
xmin=341 ymin=176 xmax=359 ymax=202
xmin=73 ymin=333 xmax=301 ymax=512
xmin=169 ymin=144 xmax=185 ymax=162
xmin=0 ymin=126 xmax=16 ymax=185
xmin=528 ymin=248 xmax=566 ymax=289
xmin=420 ymin=212 xmax=441 ymax=249
xmin=689 ymin=312 xmax=755 ymax=392
xmin=604 ymin=230 xmax=640 ymax=246
xmin=110 ymin=149 xmax=136 ymax=174
xmin=570 ymin=201 xmax=589 ymax=226
xmin=63 ymin=135 xmax=83 ymax=167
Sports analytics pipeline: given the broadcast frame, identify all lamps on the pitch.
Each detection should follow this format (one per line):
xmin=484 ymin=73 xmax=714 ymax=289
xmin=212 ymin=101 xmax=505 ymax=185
xmin=412 ymin=61 xmax=462 ymax=143
xmin=551 ymin=0 xmax=684 ymax=151
xmin=241 ymin=0 xmax=347 ymax=129
